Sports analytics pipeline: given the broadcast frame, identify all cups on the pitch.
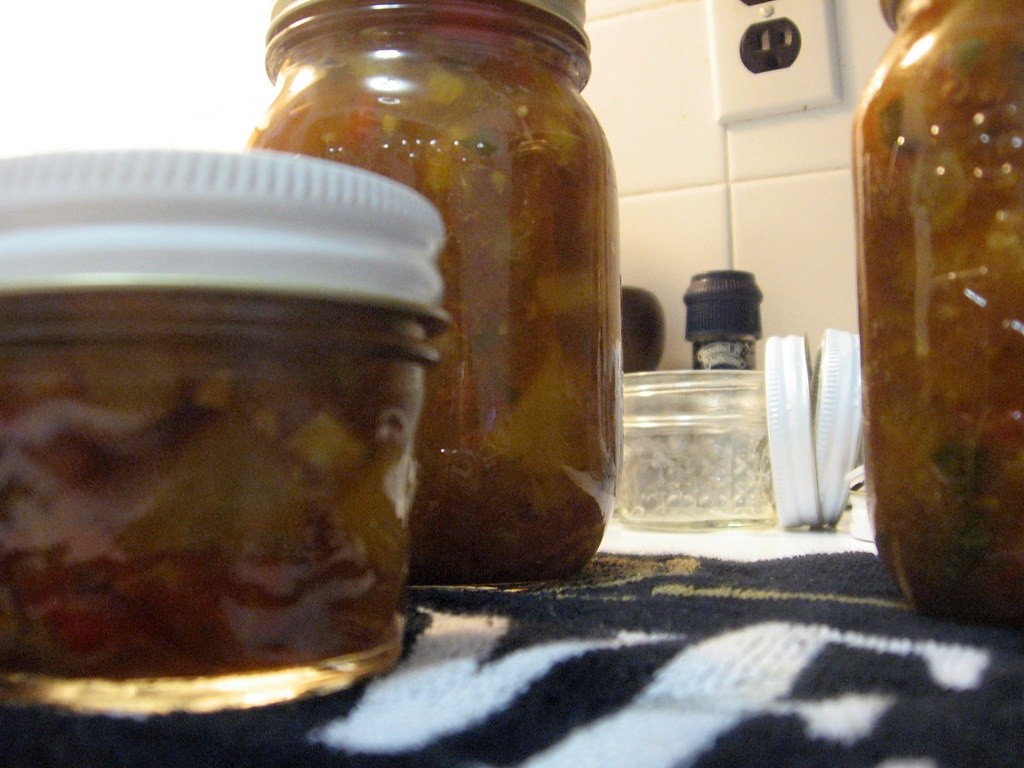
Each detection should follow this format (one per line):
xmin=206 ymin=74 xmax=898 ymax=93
xmin=608 ymin=366 xmax=777 ymax=532
xmin=0 ymin=147 xmax=457 ymax=731
xmin=245 ymin=0 xmax=623 ymax=584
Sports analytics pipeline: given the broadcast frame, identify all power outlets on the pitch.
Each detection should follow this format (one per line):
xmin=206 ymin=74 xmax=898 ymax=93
xmin=705 ymin=0 xmax=843 ymax=126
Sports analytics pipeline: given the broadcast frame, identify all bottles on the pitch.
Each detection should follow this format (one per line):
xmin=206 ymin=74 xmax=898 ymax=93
xmin=682 ymin=270 xmax=764 ymax=369
xmin=851 ymin=0 xmax=1024 ymax=628
